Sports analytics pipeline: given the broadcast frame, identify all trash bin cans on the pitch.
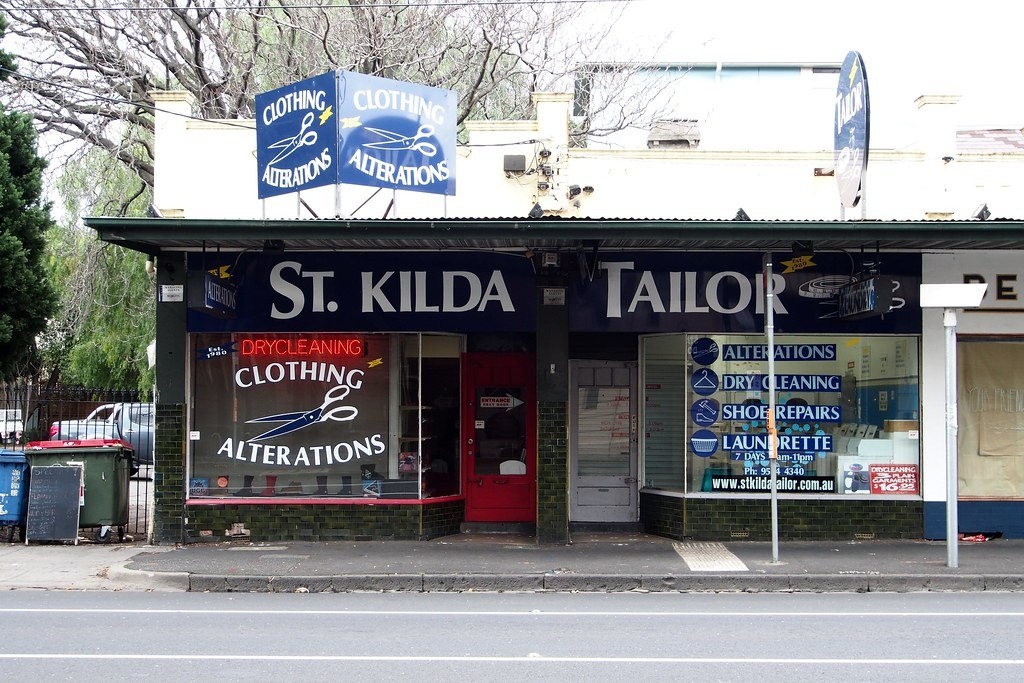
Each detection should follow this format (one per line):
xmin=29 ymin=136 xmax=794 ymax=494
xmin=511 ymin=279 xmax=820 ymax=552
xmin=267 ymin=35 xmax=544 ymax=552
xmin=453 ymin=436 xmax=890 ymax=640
xmin=0 ymin=450 xmax=30 ymax=540
xmin=26 ymin=439 xmax=133 ymax=543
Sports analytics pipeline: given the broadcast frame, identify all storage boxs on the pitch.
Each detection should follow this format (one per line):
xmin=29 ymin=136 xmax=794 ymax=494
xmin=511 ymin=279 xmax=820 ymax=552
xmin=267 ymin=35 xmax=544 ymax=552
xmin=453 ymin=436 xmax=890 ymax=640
xmin=883 ymin=418 xmax=919 ymax=431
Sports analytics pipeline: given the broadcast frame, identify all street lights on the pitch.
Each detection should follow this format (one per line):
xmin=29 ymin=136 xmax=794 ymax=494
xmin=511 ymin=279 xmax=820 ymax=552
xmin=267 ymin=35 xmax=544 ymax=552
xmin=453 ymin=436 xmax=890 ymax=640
xmin=921 ymin=282 xmax=987 ymax=571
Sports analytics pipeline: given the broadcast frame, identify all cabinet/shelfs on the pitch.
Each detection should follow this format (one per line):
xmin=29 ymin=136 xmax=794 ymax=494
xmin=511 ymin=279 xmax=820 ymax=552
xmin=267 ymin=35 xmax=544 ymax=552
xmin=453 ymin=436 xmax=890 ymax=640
xmin=397 ymin=405 xmax=432 ymax=473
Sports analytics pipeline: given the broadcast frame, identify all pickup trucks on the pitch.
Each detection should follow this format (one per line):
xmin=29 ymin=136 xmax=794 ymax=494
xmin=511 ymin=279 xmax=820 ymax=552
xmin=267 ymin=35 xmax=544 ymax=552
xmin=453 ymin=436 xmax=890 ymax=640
xmin=50 ymin=403 xmax=154 ymax=477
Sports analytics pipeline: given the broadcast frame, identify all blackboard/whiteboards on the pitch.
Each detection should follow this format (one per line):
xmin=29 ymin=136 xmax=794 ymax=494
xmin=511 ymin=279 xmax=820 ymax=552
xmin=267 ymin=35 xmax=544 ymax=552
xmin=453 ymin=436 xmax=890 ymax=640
xmin=25 ymin=465 xmax=83 ymax=540
